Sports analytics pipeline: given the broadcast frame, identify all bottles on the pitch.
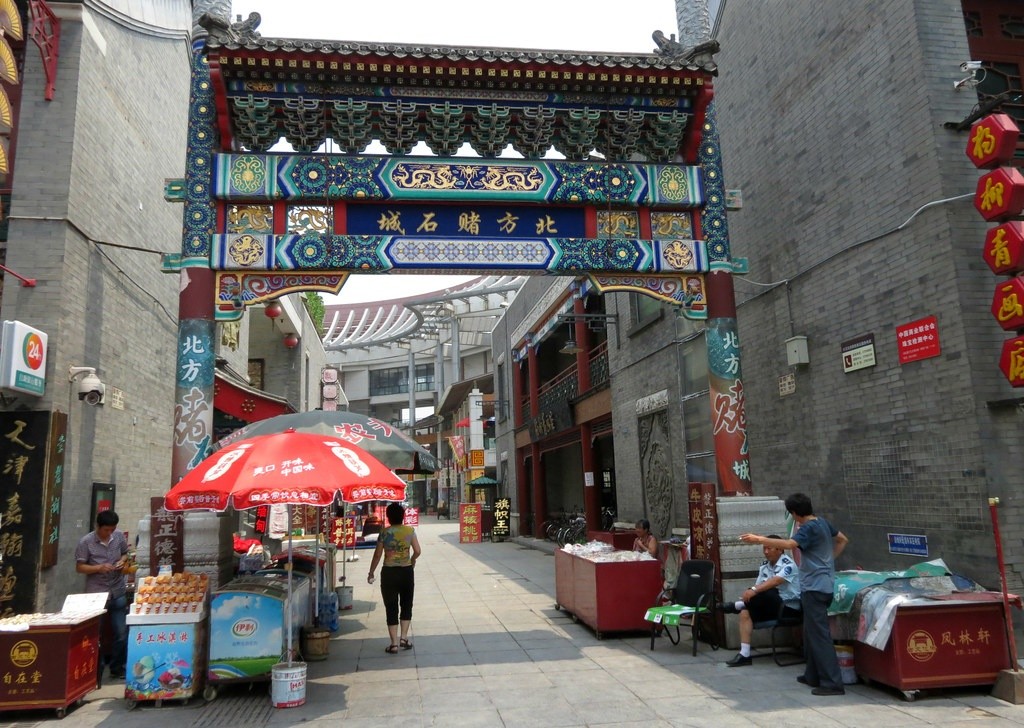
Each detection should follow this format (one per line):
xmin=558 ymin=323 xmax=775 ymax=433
xmin=240 ymin=544 xmax=271 ymax=571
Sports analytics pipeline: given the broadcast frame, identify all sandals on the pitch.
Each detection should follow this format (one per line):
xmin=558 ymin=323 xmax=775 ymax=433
xmin=385 ymin=644 xmax=399 ymax=654
xmin=400 ymin=638 xmax=413 ymax=649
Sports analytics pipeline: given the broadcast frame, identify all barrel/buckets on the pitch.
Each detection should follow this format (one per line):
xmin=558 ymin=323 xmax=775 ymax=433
xmin=270 ymin=662 xmax=308 ymax=708
xmin=314 ymin=593 xmax=339 ymax=632
xmin=834 ymin=645 xmax=857 ymax=683
xmin=302 ymin=627 xmax=330 ymax=660
xmin=336 ymin=586 xmax=354 ymax=610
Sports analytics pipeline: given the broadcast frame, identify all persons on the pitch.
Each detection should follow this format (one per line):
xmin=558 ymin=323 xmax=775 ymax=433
xmin=741 ymin=493 xmax=847 ymax=695
xmin=632 ymin=519 xmax=658 ymax=558
xmin=368 ymin=503 xmax=421 ymax=654
xmin=75 ymin=510 xmax=130 ymax=679
xmin=716 ymin=534 xmax=802 ymax=666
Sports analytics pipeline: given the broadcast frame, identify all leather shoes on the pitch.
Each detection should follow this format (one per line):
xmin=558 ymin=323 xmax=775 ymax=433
xmin=716 ymin=601 xmax=741 ymax=614
xmin=726 ymin=653 xmax=753 ymax=667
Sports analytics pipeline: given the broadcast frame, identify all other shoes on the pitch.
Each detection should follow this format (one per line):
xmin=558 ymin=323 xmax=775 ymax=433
xmin=108 ymin=667 xmax=126 ymax=679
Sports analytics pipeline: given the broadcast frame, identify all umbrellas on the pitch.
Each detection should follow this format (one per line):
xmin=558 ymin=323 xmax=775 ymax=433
xmin=164 ymin=407 xmax=435 ymax=667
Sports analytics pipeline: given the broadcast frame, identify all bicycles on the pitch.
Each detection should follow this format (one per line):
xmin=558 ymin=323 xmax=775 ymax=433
xmin=544 ymin=504 xmax=619 ymax=550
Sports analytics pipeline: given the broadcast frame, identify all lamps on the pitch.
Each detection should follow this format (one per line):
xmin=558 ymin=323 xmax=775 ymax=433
xmin=557 ymin=312 xmax=620 ymax=354
xmin=476 ymin=400 xmax=510 ymax=421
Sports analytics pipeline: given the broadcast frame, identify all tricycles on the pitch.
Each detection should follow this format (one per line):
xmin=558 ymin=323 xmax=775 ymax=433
xmin=437 ymin=506 xmax=450 ymax=520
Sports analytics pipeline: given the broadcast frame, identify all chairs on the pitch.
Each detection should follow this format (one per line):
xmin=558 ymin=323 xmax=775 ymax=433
xmin=650 ymin=559 xmax=721 ymax=656
xmin=750 ymin=599 xmax=805 ymax=667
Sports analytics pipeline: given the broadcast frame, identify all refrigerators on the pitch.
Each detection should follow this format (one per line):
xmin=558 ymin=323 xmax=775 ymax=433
xmin=202 ymin=569 xmax=311 ymax=701
xmin=278 ymin=547 xmax=327 ymax=627
xmin=304 ymin=544 xmax=336 ymax=593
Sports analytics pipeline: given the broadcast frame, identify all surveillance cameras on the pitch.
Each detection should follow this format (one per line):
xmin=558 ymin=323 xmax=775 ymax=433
xmin=959 ymin=61 xmax=982 ymax=72
xmin=77 ymin=374 xmax=104 ymax=405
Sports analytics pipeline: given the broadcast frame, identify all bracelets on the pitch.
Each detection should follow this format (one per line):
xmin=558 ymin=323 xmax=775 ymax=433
xmin=369 ymin=573 xmax=373 ymax=576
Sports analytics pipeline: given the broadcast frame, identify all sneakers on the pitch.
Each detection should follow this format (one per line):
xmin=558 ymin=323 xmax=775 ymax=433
xmin=797 ymin=676 xmax=820 ymax=687
xmin=812 ymin=685 xmax=845 ymax=695
